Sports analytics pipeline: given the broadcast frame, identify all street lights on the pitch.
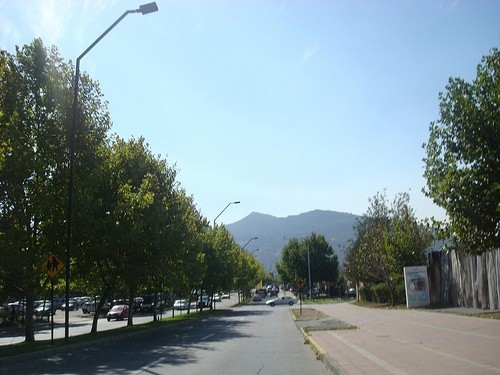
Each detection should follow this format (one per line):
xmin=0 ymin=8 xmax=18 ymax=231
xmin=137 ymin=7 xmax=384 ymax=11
xmin=63 ymin=2 xmax=159 ymax=334
xmin=214 ymin=201 xmax=241 ymax=309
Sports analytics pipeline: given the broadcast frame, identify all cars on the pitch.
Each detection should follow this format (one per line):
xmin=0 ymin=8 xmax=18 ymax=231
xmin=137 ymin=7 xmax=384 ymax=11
xmin=266 ymin=295 xmax=298 ymax=307
xmin=252 ymin=284 xmax=278 ymax=302
xmin=197 ymin=296 xmax=211 ymax=307
xmin=173 ymin=299 xmax=197 ymax=310
xmin=345 ymin=288 xmax=356 ymax=297
xmin=106 ymin=305 xmax=129 ymax=321
xmin=0 ymin=299 xmax=55 ymax=316
xmin=221 ymin=291 xmax=230 ymax=299
xmin=61 ymin=296 xmax=101 ymax=314
xmin=213 ymin=293 xmax=221 ymax=302
